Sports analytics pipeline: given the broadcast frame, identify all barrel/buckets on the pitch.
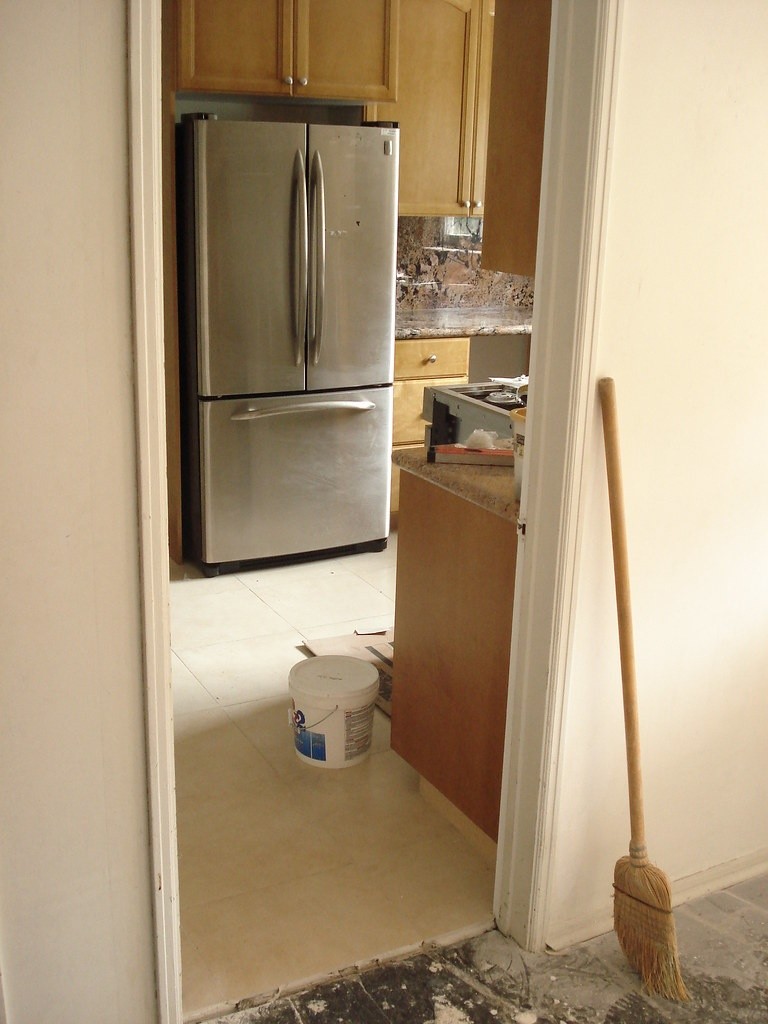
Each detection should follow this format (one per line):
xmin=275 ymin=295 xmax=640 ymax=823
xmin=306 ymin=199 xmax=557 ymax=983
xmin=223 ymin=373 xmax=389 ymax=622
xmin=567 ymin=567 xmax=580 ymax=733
xmin=287 ymin=655 xmax=379 ymax=768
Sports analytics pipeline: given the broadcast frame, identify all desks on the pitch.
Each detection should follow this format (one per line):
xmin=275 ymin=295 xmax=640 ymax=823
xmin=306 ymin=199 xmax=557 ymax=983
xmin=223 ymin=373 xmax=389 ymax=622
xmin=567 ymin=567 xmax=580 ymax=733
xmin=391 ymin=445 xmax=516 ymax=846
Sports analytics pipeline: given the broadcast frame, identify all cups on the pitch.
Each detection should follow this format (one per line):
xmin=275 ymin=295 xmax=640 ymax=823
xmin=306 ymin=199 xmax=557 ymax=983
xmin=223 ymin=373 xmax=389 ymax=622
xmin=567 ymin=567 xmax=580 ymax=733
xmin=509 ymin=407 xmax=527 ymax=502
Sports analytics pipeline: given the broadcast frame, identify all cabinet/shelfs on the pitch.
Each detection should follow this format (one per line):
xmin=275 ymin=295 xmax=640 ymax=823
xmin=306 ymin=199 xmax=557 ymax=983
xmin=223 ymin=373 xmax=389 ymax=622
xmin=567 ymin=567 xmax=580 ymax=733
xmin=482 ymin=0 xmax=552 ymax=280
xmin=391 ymin=337 xmax=471 ymax=512
xmin=174 ymin=0 xmax=398 ymax=106
xmin=329 ymin=0 xmax=495 ymax=218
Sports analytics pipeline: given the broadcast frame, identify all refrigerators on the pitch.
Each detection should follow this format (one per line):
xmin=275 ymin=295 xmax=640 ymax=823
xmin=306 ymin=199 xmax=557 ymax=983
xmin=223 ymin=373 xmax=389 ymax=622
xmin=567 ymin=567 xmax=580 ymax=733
xmin=176 ymin=113 xmax=401 ymax=577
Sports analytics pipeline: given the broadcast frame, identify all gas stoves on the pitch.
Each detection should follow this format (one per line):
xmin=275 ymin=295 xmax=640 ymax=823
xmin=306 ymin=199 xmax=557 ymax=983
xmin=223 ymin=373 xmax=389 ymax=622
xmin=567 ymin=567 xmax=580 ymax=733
xmin=422 ymin=378 xmax=527 ymax=447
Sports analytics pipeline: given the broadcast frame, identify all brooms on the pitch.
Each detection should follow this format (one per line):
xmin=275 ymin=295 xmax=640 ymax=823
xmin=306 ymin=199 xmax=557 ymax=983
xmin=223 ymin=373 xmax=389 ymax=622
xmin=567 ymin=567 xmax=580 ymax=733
xmin=595 ymin=377 xmax=695 ymax=1005
